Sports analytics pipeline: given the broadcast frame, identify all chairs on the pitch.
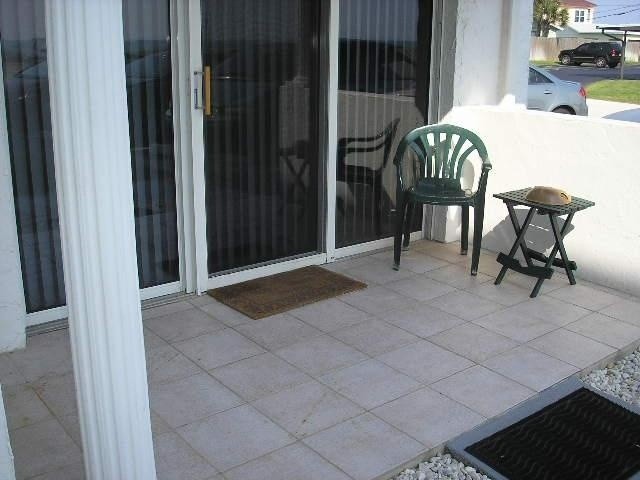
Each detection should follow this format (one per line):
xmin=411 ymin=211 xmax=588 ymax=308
xmin=393 ymin=123 xmax=492 ymax=275
xmin=337 ymin=118 xmax=400 ymax=236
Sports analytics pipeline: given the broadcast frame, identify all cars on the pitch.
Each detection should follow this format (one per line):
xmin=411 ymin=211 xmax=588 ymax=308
xmin=559 ymin=41 xmax=625 ymax=68
xmin=527 ymin=65 xmax=588 ymax=116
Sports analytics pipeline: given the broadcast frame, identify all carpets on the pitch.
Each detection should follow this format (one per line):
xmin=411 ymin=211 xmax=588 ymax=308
xmin=447 ymin=376 xmax=640 ymax=480
xmin=206 ymin=265 xmax=367 ymax=320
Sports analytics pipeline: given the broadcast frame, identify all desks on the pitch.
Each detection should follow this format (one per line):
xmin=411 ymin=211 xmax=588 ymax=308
xmin=494 ymin=187 xmax=595 ymax=298
xmin=279 ymin=147 xmax=312 ymax=219
